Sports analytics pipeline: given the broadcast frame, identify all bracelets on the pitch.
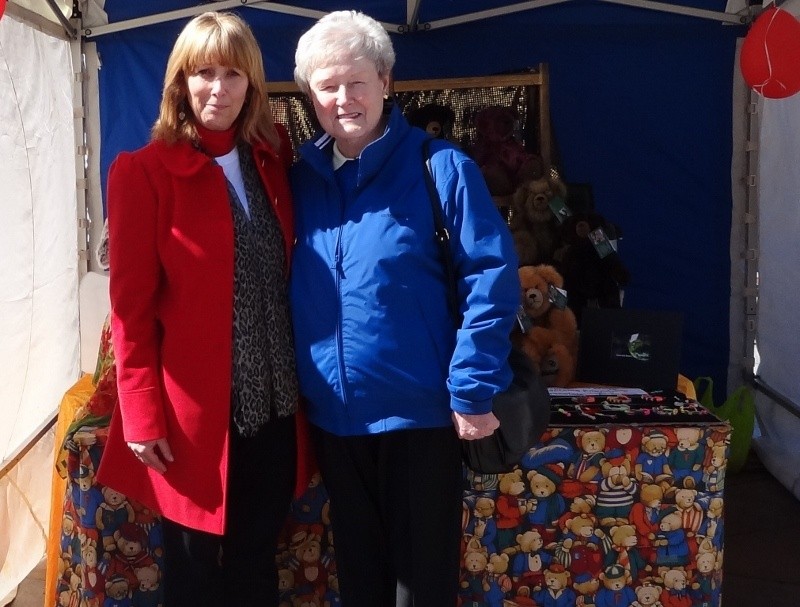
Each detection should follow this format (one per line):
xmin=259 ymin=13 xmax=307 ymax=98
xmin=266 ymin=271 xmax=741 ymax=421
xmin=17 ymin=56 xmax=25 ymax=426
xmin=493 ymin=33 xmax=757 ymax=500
xmin=559 ymin=394 xmax=709 ymax=416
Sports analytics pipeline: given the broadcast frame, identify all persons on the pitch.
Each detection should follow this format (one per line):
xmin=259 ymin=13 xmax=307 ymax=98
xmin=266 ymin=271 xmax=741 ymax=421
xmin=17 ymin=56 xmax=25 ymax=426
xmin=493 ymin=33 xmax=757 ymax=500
xmin=288 ymin=11 xmax=522 ymax=607
xmin=96 ymin=12 xmax=315 ymax=607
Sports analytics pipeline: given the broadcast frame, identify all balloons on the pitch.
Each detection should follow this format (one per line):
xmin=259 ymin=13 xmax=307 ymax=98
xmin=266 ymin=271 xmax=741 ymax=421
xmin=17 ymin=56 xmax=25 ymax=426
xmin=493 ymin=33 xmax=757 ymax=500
xmin=740 ymin=7 xmax=800 ymax=99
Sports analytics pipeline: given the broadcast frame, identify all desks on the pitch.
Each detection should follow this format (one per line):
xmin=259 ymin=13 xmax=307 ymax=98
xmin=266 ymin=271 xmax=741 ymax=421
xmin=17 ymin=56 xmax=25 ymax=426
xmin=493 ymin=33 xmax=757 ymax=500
xmin=55 ymin=384 xmax=733 ymax=607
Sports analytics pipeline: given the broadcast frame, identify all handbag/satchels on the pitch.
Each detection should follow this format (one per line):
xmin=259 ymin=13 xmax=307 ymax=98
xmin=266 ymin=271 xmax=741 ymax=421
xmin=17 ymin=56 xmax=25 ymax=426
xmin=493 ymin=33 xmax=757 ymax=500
xmin=460 ymin=339 xmax=550 ymax=475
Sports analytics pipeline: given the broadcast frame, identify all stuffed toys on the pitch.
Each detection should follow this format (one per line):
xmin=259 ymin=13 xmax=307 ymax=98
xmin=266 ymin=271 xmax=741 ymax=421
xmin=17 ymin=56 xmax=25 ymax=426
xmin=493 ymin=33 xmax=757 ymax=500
xmin=411 ymin=104 xmax=625 ymax=386
